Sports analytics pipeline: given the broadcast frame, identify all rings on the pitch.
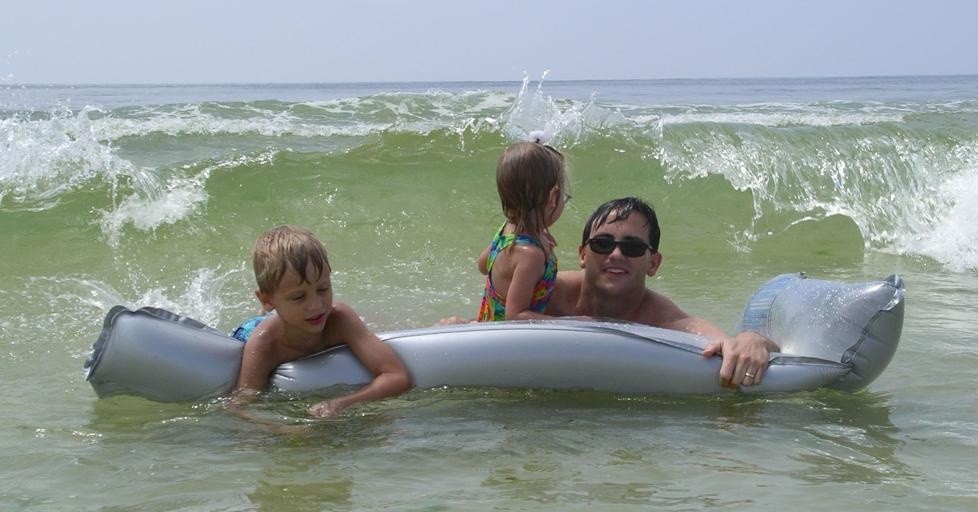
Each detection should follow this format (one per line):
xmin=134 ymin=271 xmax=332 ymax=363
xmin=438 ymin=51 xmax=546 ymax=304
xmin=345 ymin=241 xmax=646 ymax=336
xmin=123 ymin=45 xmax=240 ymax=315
xmin=744 ymin=371 xmax=755 ymax=380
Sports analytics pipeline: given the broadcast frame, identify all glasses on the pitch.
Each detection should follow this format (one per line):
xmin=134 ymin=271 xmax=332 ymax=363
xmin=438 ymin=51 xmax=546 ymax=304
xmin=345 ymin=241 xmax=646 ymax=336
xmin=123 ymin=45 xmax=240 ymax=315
xmin=555 ymin=186 xmax=575 ymax=206
xmin=577 ymin=232 xmax=658 ymax=256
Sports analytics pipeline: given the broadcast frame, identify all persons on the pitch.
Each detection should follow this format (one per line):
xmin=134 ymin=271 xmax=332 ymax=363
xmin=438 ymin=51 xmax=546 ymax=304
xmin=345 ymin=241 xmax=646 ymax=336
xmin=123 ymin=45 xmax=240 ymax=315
xmin=222 ymin=226 xmax=412 ymax=434
xmin=473 ymin=139 xmax=596 ymax=321
xmin=434 ymin=196 xmax=780 ymax=399
